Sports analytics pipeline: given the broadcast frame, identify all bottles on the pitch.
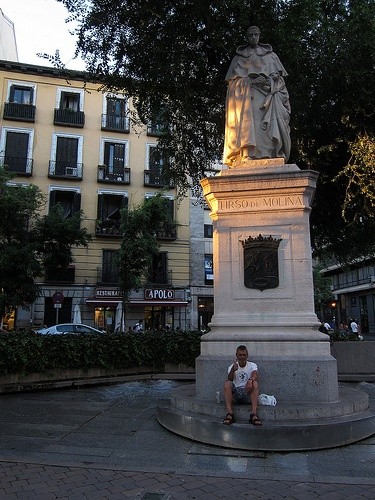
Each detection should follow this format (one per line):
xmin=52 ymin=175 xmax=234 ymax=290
xmin=216 ymin=390 xmax=221 ymax=404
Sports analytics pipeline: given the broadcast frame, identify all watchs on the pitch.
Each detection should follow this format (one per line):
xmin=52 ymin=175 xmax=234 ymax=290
xmin=248 ymin=377 xmax=253 ymax=380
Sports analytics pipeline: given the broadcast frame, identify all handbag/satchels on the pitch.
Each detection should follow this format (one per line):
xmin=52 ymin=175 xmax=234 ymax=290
xmin=257 ymin=394 xmax=277 ymax=407
xmin=358 ymin=335 xmax=363 ymax=341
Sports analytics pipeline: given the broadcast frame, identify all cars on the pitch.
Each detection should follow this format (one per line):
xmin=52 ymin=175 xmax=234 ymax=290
xmin=35 ymin=323 xmax=107 ymax=335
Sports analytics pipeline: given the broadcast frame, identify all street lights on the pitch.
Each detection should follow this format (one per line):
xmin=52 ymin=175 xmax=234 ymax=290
xmin=331 ymin=300 xmax=336 ymax=328
xmin=198 ymin=305 xmax=205 ymax=331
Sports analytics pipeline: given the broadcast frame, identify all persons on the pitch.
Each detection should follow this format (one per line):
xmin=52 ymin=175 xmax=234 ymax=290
xmin=98 ymin=312 xmax=103 ymax=326
xmin=42 ymin=325 xmax=47 ymax=329
xmin=223 ymin=345 xmax=263 ymax=426
xmin=223 ymin=26 xmax=291 ymax=166
xmin=318 ymin=317 xmax=361 ymax=339
xmin=102 ymin=327 xmax=111 ymax=335
xmin=129 ymin=317 xmax=206 ymax=332
xmin=3 ymin=314 xmax=14 ymax=330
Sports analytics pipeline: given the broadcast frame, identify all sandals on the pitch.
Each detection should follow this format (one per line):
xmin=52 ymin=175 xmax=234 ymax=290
xmin=223 ymin=413 xmax=235 ymax=425
xmin=249 ymin=413 xmax=262 ymax=426
